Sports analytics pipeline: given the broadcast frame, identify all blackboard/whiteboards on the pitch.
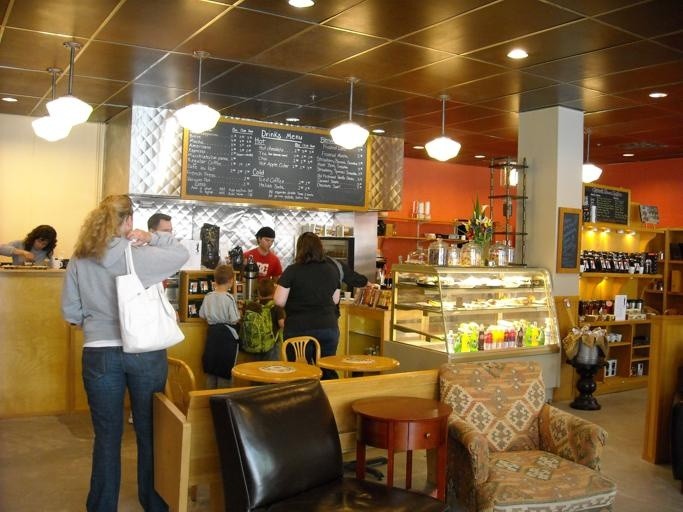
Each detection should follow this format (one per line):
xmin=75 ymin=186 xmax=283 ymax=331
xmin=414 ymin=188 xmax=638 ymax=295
xmin=556 ymin=207 xmax=582 ymax=273
xmin=582 ymin=183 xmax=631 ymax=227
xmin=179 ymin=118 xmax=371 ymax=211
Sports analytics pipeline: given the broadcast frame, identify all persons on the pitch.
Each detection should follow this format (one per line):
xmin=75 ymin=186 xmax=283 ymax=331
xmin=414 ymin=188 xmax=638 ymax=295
xmin=273 ymin=233 xmax=341 ymax=378
xmin=1 ymin=224 xmax=55 ymax=264
xmin=200 ymin=265 xmax=240 ymax=388
xmin=147 ymin=214 xmax=173 ymax=232
xmin=62 ymin=197 xmax=186 ymax=512
xmin=246 ymin=278 xmax=285 ymax=361
xmin=249 ymin=228 xmax=281 ymax=300
xmin=325 ymin=256 xmax=377 ymax=319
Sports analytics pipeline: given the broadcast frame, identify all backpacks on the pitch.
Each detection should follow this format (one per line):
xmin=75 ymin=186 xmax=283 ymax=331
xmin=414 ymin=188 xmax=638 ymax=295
xmin=239 ymin=300 xmax=283 ymax=353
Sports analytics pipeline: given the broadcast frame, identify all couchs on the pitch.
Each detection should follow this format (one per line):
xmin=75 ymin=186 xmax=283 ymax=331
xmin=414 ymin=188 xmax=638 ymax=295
xmin=437 ymin=360 xmax=613 ymax=512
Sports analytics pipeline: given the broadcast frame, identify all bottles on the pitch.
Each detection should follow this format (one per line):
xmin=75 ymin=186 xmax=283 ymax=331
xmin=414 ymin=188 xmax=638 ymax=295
xmin=629 ymin=300 xmax=636 ymax=310
xmin=625 ymin=300 xmax=629 ymax=309
xmin=448 ymin=330 xmax=454 ymax=353
xmin=628 ymin=260 xmax=635 ymax=274
xmin=648 ymin=253 xmax=657 ymax=272
xmin=485 ymin=325 xmax=504 ymax=349
xmin=454 ymin=332 xmax=462 ymax=353
xmin=471 ymin=324 xmax=478 ymax=352
xmin=605 ymin=260 xmax=611 ymax=270
xmin=591 ymin=300 xmax=597 ymax=314
xmin=517 ymin=325 xmax=523 ymax=347
xmin=586 ymin=302 xmax=592 ymax=315
xmin=618 ymin=260 xmax=623 ymax=272
xmin=545 ymin=323 xmax=551 ymax=347
xmin=644 ymin=257 xmax=652 ymax=274
xmin=600 ymin=259 xmax=605 ymax=271
xmin=636 ymin=300 xmax=642 ymax=314
xmin=579 ymin=258 xmax=584 ymax=272
xmin=461 ymin=324 xmax=471 ymax=352
xmin=509 ymin=323 xmax=515 ymax=348
xmin=640 ymin=255 xmax=645 ymax=267
xmin=504 ymin=324 xmax=509 ymax=348
xmin=634 ymin=257 xmax=639 ymax=273
xmin=539 ymin=326 xmax=545 ymax=346
xmin=613 ymin=259 xmax=619 ymax=271
xmin=623 ymin=256 xmax=628 ymax=271
xmin=532 ymin=321 xmax=539 ymax=347
xmin=581 ymin=301 xmax=587 ymax=315
xmin=525 ymin=322 xmax=532 ymax=346
xmin=599 ymin=300 xmax=605 ymax=314
xmin=580 ymin=250 xmax=642 ymax=260
xmin=589 ymin=258 xmax=595 ymax=271
xmin=595 ymin=258 xmax=601 ymax=271
xmin=606 ymin=300 xmax=614 ymax=315
xmin=583 ymin=258 xmax=589 ymax=272
xmin=479 ymin=322 xmax=485 ymax=351
xmin=578 ymin=301 xmax=581 ymax=316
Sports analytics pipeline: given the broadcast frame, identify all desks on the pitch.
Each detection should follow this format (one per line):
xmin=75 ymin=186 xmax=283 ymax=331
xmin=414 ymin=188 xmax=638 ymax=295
xmin=351 ymin=396 xmax=454 ymax=505
xmin=230 ymin=361 xmax=323 ymax=390
xmin=1 ymin=266 xmax=92 ymax=417
xmin=171 ymin=322 xmax=260 ymax=389
xmin=317 ymin=355 xmax=401 ymax=379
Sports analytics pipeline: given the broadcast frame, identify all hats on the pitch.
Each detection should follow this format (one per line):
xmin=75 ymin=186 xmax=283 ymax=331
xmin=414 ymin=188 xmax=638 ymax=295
xmin=255 ymin=227 xmax=274 ymax=237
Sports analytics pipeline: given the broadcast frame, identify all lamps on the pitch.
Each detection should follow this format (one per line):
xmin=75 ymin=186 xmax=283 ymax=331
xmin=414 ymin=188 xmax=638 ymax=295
xmin=44 ymin=42 xmax=95 ymax=130
xmin=173 ymin=51 xmax=221 ymax=136
xmin=424 ymin=93 xmax=462 ymax=162
xmin=31 ymin=66 xmax=73 ymax=145
xmin=582 ymin=130 xmax=603 ymax=184
xmin=328 ymin=77 xmax=370 ymax=153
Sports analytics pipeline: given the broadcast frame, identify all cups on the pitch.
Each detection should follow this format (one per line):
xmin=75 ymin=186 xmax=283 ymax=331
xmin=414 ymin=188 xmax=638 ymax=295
xmin=344 ymin=291 xmax=351 ymax=299
xmin=50 ymin=257 xmax=62 ymax=269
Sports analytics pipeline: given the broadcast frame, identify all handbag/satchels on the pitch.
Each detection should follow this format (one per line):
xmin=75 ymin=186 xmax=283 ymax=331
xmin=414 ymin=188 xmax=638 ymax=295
xmin=115 ymin=240 xmax=186 ymax=354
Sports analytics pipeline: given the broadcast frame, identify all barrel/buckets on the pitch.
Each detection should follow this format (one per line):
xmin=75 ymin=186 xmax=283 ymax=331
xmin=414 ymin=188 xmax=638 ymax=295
xmin=577 ymin=336 xmax=599 ymax=365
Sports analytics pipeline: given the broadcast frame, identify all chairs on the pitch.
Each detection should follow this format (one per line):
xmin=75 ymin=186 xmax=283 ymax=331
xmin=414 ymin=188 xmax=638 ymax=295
xmin=209 ymin=378 xmax=451 ymax=512
xmin=281 ymin=334 xmax=321 ymax=371
xmin=164 ymin=357 xmax=196 ymax=416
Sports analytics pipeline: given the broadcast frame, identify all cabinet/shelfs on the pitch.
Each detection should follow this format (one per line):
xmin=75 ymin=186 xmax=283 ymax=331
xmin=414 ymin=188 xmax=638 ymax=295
xmin=578 ymin=222 xmax=666 ymax=398
xmin=377 ymin=216 xmax=457 ymax=279
xmin=388 ymin=263 xmax=562 ymax=404
xmin=345 ymin=304 xmax=430 ymax=379
xmin=666 ymin=229 xmax=683 ymax=315
xmin=162 ymin=269 xmax=248 ymax=324
